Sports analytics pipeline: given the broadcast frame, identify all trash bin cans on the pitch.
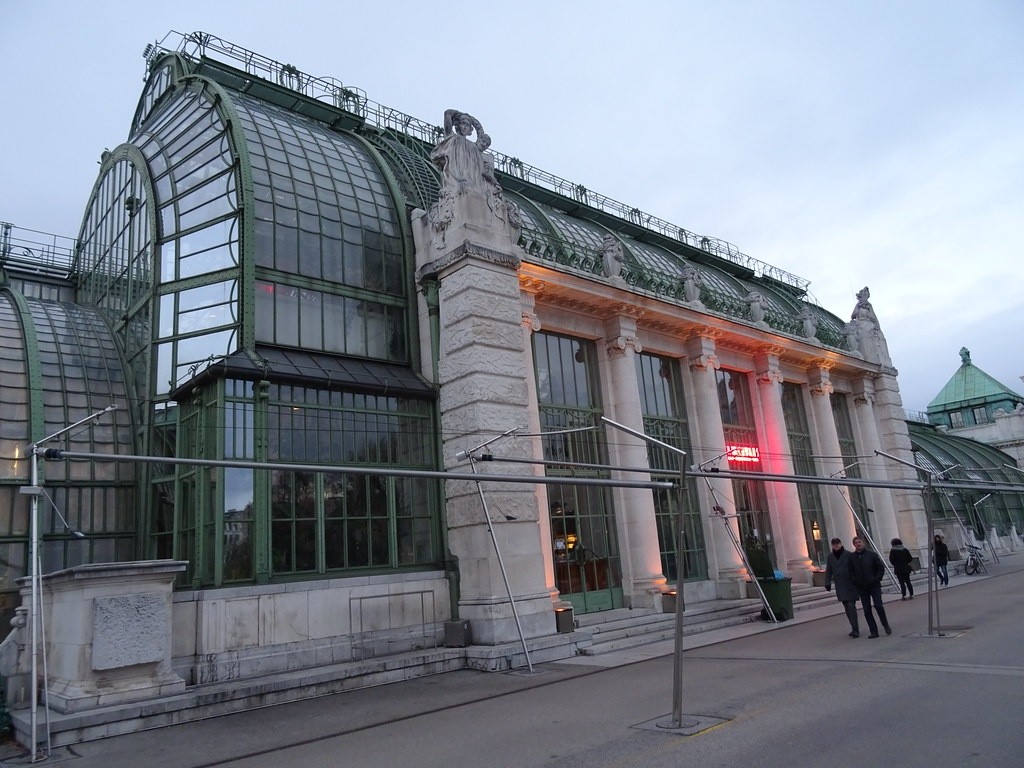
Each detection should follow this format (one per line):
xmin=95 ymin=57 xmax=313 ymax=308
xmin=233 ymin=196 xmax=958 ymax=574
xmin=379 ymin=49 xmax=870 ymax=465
xmin=758 ymin=577 xmax=794 ymax=621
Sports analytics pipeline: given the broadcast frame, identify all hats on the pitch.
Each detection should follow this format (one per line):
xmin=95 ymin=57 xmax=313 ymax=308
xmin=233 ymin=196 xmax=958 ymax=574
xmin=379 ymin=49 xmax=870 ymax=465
xmin=832 ymin=538 xmax=841 ymax=545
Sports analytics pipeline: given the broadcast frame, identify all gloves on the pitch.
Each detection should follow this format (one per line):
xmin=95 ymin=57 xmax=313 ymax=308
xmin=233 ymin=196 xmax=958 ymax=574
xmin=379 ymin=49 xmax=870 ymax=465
xmin=825 ymin=585 xmax=831 ymax=592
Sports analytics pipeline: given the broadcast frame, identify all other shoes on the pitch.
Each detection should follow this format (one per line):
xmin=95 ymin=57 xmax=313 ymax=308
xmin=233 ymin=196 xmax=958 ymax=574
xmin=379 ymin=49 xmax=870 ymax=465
xmin=910 ymin=594 xmax=913 ymax=599
xmin=849 ymin=631 xmax=859 ymax=638
xmin=885 ymin=625 xmax=891 ymax=635
xmin=945 ymin=578 xmax=948 ymax=585
xmin=868 ymin=634 xmax=879 ymax=638
xmin=902 ymin=596 xmax=906 ymax=600
xmin=940 ymin=578 xmax=944 ymax=584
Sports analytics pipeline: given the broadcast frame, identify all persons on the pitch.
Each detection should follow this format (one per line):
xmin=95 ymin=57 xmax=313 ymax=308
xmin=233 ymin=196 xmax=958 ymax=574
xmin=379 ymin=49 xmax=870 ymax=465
xmin=848 ymin=537 xmax=892 ymax=639
xmin=824 ymin=538 xmax=860 ymax=638
xmin=932 ymin=533 xmax=950 ymax=585
xmin=430 ymin=109 xmax=501 ymax=197
xmin=889 ymin=538 xmax=914 ymax=600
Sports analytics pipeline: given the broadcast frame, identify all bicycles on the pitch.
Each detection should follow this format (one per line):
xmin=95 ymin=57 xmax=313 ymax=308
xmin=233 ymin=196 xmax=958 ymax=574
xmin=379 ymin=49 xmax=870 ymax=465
xmin=964 ymin=542 xmax=985 ymax=576
